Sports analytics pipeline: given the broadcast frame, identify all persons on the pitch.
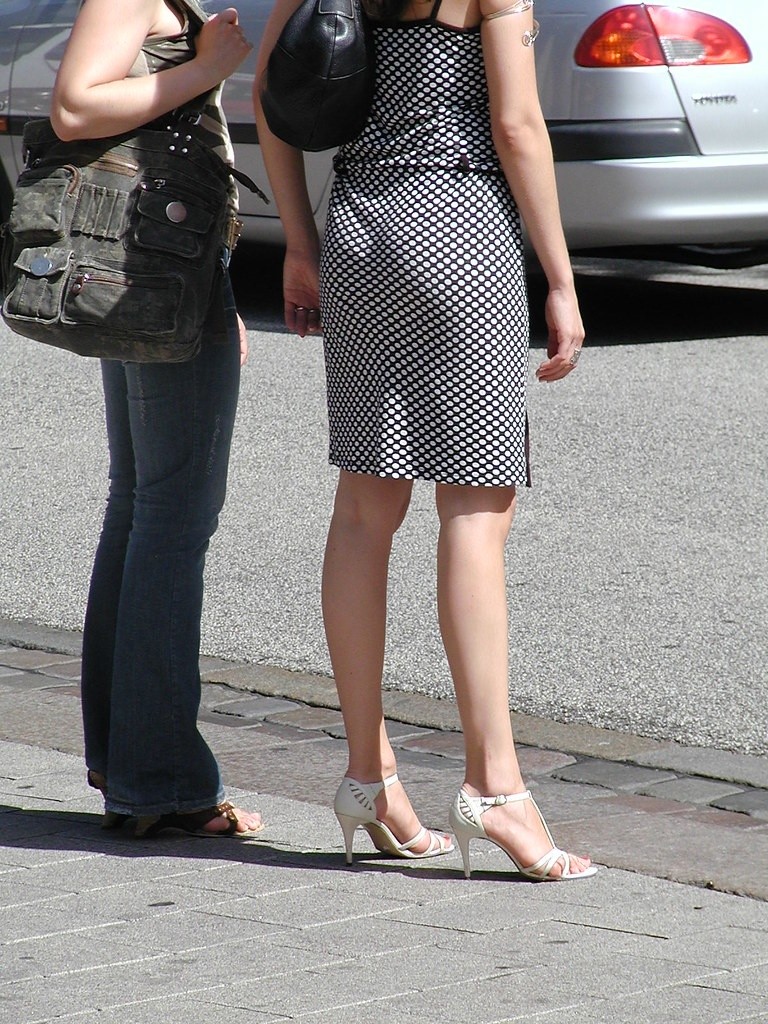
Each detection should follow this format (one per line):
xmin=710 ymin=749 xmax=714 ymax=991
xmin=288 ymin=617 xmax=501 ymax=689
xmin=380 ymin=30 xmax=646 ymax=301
xmin=252 ymin=0 xmax=599 ymax=880
xmin=50 ymin=0 xmax=265 ymax=837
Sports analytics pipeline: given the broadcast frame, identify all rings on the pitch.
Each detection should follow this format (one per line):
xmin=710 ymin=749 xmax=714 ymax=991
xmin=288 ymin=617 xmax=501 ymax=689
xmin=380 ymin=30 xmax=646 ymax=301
xmin=296 ymin=307 xmax=307 ymax=311
xmin=310 ymin=309 xmax=316 ymax=313
xmin=571 ymin=349 xmax=582 ymax=362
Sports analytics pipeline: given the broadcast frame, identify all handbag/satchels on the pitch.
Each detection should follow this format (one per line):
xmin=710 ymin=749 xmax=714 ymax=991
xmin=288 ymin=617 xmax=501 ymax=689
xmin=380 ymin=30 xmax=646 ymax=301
xmin=259 ymin=0 xmax=375 ymax=152
xmin=1 ymin=0 xmax=271 ymax=365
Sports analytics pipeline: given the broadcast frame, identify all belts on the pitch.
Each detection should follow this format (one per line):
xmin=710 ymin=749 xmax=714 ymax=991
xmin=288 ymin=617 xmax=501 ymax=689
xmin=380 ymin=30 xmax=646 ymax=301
xmin=223 ymin=218 xmax=244 ymax=250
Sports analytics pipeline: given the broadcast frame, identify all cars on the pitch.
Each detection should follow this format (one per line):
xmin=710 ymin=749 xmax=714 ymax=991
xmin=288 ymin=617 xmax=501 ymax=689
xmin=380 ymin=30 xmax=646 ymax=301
xmin=0 ymin=1 xmax=768 ymax=270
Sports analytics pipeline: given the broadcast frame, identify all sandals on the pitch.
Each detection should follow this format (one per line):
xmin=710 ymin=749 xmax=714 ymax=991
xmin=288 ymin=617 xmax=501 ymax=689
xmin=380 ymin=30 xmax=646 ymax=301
xmin=134 ymin=800 xmax=265 ymax=840
xmin=86 ymin=768 xmax=135 ymax=831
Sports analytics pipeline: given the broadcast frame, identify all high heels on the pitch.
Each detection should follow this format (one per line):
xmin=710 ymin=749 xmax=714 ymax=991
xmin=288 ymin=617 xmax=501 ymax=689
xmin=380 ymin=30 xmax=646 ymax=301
xmin=332 ymin=772 xmax=455 ymax=865
xmin=448 ymin=789 xmax=600 ymax=881
